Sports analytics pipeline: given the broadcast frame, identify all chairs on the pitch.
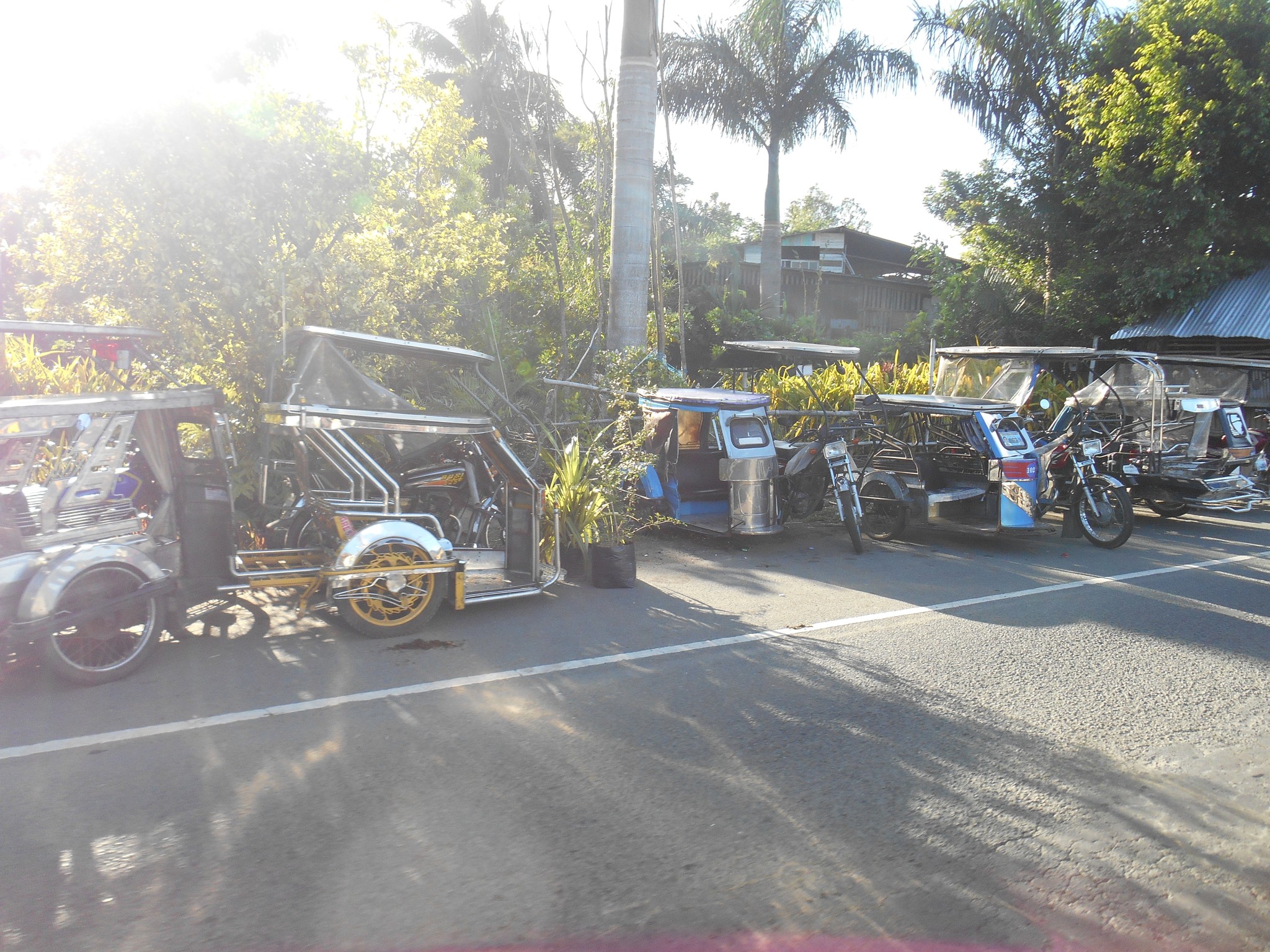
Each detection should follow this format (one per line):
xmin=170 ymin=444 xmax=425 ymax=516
xmin=0 ymin=491 xmax=38 ymax=553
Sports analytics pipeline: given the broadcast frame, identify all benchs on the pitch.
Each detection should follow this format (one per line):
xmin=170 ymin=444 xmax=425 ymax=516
xmin=879 ymin=451 xmax=984 ymax=518
xmin=674 ymin=448 xmax=732 ymax=515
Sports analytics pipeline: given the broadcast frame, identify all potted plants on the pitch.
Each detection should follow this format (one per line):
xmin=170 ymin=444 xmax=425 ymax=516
xmin=541 ymin=344 xmax=688 ymax=591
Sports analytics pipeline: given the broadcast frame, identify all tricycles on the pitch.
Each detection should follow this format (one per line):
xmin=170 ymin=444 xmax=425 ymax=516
xmin=694 ymin=339 xmax=890 ymax=557
xmin=925 ymin=338 xmax=1142 ymax=551
xmin=1065 ymin=351 xmax=1262 ymax=521
xmin=0 ymin=311 xmax=788 ymax=691
